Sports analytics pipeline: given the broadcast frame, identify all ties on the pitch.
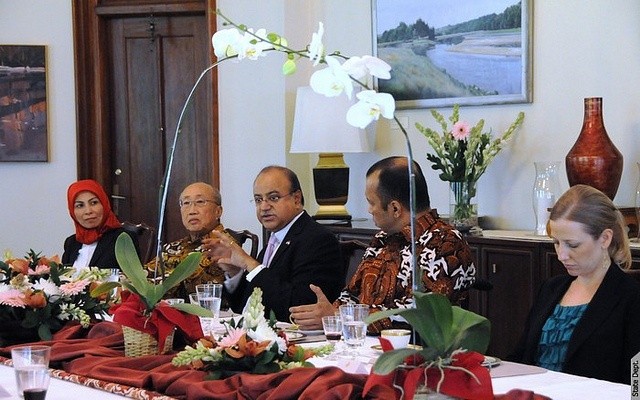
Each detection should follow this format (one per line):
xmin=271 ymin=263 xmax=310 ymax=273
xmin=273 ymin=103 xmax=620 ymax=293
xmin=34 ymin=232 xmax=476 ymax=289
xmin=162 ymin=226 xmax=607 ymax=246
xmin=261 ymin=235 xmax=277 ymax=266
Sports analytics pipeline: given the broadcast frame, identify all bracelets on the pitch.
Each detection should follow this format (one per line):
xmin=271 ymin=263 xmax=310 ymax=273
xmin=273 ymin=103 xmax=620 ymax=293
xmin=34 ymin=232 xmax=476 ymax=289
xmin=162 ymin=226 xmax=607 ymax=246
xmin=242 ymin=258 xmax=260 ymax=276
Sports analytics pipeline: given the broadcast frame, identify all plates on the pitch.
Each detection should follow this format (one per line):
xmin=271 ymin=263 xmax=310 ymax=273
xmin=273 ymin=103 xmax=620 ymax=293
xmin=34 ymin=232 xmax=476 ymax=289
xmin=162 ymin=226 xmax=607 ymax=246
xmin=370 ymin=344 xmax=424 ymax=352
xmin=278 ymin=331 xmax=307 ymax=341
xmin=478 ymin=356 xmax=501 ymax=366
xmin=336 ymin=336 xmax=378 ymax=348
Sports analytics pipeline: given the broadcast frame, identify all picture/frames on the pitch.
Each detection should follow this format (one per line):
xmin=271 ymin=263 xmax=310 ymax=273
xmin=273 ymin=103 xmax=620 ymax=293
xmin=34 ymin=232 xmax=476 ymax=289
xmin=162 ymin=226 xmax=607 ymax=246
xmin=0 ymin=44 xmax=51 ymax=161
xmin=370 ymin=0 xmax=535 ymax=110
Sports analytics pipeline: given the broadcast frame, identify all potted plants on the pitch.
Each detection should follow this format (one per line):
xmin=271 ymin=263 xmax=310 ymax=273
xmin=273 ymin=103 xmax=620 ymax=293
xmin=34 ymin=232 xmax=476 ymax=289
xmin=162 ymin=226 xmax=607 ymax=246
xmin=93 ymin=233 xmax=209 ymax=362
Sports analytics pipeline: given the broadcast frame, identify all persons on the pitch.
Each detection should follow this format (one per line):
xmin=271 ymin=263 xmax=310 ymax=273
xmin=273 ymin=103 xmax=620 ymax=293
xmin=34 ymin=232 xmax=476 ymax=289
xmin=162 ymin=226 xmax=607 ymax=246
xmin=60 ymin=179 xmax=144 ymax=268
xmin=289 ymin=156 xmax=476 ymax=346
xmin=143 ymin=182 xmax=239 ymax=301
xmin=199 ymin=165 xmax=342 ymax=324
xmin=514 ymin=183 xmax=636 ymax=385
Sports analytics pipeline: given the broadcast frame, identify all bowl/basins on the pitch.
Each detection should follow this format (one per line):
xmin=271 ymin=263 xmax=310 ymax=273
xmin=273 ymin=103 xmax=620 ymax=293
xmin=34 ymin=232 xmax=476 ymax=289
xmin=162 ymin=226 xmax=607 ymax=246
xmin=380 ymin=328 xmax=411 ymax=349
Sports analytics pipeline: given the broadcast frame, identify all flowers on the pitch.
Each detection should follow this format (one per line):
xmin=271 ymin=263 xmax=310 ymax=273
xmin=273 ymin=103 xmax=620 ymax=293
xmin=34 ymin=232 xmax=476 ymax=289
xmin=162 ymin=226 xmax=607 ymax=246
xmin=0 ymin=249 xmax=122 ymax=343
xmin=211 ymin=9 xmax=418 ymax=305
xmin=172 ymin=287 xmax=336 ymax=382
xmin=157 ymin=44 xmax=391 ymax=285
xmin=415 ymin=104 xmax=524 ymax=181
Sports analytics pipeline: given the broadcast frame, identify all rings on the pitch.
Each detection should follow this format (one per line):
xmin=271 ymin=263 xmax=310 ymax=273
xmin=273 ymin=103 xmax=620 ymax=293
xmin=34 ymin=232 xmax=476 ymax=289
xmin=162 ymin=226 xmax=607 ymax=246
xmin=226 ymin=239 xmax=237 ymax=249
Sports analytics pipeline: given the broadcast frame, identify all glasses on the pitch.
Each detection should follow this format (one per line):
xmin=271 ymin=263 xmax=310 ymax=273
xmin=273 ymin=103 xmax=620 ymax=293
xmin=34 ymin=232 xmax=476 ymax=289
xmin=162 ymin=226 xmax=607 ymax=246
xmin=248 ymin=190 xmax=296 ymax=205
xmin=179 ymin=198 xmax=220 ymax=208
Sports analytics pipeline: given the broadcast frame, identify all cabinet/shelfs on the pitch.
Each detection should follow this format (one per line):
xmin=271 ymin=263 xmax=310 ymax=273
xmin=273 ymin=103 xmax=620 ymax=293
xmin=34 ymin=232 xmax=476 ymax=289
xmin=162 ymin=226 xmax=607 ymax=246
xmin=539 ymin=241 xmax=640 ymax=350
xmin=335 ymin=230 xmax=374 ymax=291
xmin=462 ymin=240 xmax=537 ymax=366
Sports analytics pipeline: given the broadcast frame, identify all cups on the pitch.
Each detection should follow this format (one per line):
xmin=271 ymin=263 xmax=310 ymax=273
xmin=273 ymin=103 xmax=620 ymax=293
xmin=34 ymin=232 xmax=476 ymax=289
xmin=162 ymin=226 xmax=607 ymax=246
xmin=19 ymin=365 xmax=52 ymax=399
xmin=339 ymin=304 xmax=368 ymax=345
xmin=195 ymin=283 xmax=222 ymax=318
xmin=189 ymin=293 xmax=197 ymax=304
xmin=320 ymin=314 xmax=340 ymax=339
xmin=10 ymin=346 xmax=49 ymax=391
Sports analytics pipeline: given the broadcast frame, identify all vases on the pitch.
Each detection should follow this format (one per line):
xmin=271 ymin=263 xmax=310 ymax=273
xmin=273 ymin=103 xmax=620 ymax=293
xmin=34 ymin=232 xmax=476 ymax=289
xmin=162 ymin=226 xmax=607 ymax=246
xmin=449 ymin=180 xmax=479 ymax=230
xmin=532 ymin=162 xmax=563 ymax=237
xmin=564 ymin=96 xmax=623 ymax=202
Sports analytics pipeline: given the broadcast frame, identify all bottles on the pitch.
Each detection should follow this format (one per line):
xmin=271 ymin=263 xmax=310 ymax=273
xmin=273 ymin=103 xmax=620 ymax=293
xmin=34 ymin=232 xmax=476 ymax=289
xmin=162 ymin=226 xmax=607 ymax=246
xmin=166 ymin=299 xmax=185 ymax=305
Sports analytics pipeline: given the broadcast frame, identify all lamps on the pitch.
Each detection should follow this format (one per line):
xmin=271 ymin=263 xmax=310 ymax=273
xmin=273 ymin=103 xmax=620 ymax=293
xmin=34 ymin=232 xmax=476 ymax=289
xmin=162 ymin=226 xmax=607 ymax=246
xmin=289 ymin=87 xmax=379 ymax=227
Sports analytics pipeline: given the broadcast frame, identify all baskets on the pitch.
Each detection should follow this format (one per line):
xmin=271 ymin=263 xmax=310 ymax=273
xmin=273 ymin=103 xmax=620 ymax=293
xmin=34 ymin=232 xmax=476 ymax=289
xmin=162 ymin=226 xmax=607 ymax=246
xmin=121 ymin=323 xmax=176 ymax=358
